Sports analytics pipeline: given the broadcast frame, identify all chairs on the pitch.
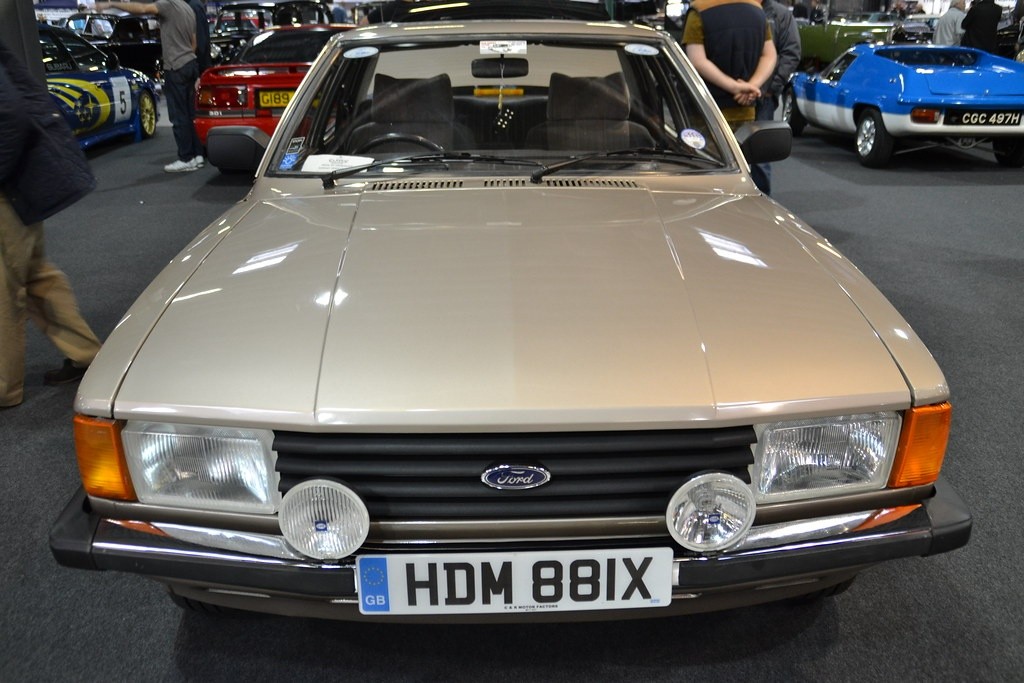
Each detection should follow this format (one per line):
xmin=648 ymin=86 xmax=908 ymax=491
xmin=526 ymin=69 xmax=658 ymax=152
xmin=349 ymin=72 xmax=479 ymax=159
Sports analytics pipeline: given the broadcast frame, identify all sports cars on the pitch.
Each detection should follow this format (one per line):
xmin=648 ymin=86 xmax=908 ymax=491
xmin=781 ymin=42 xmax=1024 ymax=167
xmin=39 ymin=21 xmax=162 ymax=151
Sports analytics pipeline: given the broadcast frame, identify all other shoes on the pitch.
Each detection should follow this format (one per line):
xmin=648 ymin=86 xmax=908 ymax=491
xmin=44 ymin=359 xmax=87 ymax=386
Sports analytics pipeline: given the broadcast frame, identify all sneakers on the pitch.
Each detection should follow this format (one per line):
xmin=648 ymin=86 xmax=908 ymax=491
xmin=164 ymin=158 xmax=198 ymax=173
xmin=195 ymin=155 xmax=204 ymax=168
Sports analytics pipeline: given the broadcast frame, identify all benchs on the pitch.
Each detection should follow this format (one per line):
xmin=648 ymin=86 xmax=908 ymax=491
xmin=356 ymin=94 xmax=642 ymax=149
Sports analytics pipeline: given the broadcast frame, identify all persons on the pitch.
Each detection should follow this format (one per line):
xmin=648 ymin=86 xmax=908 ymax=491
xmin=682 ymin=1 xmax=801 ymax=196
xmin=88 ymin=0 xmax=212 ymax=171
xmin=0 ymin=0 xmax=103 ymax=408
xmin=889 ymin=0 xmax=1024 ymax=55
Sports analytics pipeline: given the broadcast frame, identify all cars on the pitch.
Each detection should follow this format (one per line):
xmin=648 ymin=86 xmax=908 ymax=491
xmin=48 ymin=17 xmax=974 ymax=651
xmin=56 ymin=0 xmax=945 ymax=179
